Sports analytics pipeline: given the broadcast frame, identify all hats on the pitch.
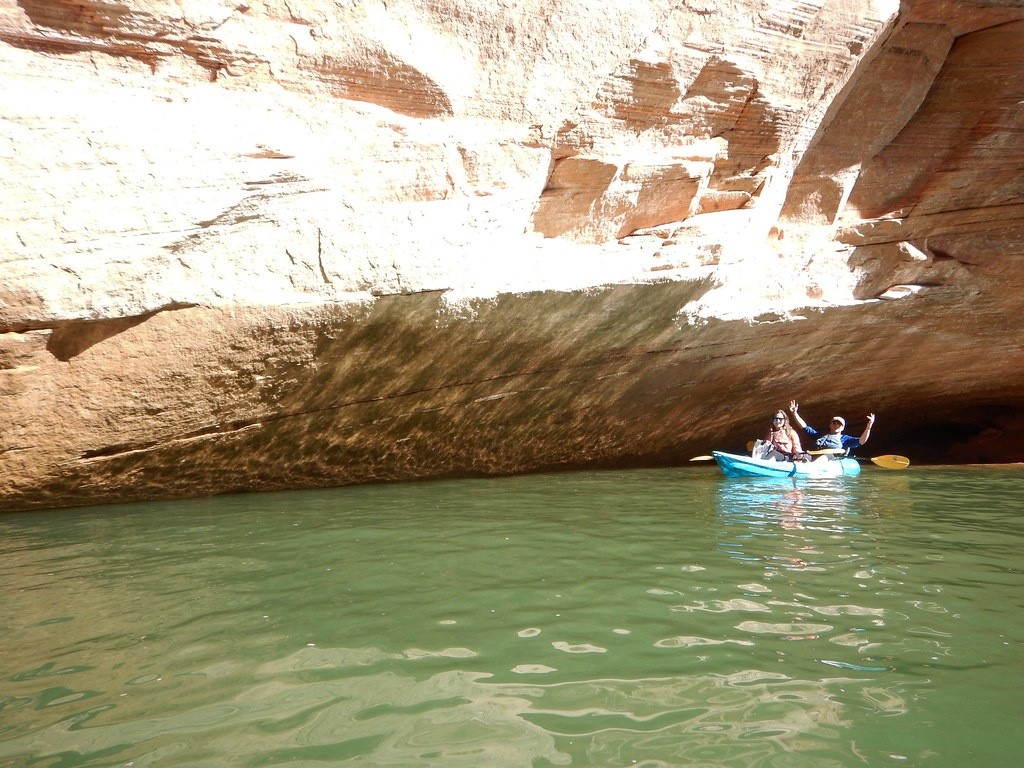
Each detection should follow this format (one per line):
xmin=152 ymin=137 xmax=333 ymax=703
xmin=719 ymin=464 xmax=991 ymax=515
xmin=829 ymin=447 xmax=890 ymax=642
xmin=832 ymin=416 xmax=846 ymax=426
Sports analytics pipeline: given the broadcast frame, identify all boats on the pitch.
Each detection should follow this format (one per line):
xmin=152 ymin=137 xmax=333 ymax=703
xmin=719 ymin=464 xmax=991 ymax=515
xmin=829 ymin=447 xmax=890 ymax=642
xmin=713 ymin=451 xmax=861 ymax=479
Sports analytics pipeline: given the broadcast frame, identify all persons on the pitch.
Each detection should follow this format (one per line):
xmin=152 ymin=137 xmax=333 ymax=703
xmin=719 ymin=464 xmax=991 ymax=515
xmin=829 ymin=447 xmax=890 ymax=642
xmin=790 ymin=400 xmax=874 ymax=463
xmin=766 ymin=410 xmax=803 ymax=461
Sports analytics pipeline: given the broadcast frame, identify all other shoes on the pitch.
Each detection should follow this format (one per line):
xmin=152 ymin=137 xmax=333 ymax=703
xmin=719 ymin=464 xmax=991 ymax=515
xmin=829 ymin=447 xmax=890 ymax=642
xmin=802 ymin=458 xmax=809 ymax=463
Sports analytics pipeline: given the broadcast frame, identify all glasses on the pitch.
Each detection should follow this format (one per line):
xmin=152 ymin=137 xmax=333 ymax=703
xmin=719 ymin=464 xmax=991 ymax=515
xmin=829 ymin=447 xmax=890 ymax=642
xmin=773 ymin=418 xmax=785 ymax=422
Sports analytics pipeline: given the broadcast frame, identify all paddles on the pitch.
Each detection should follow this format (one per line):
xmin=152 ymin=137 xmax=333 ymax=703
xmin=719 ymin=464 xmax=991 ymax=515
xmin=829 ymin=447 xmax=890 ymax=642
xmin=688 ymin=448 xmax=848 ymax=462
xmin=746 ymin=439 xmax=911 ymax=471
